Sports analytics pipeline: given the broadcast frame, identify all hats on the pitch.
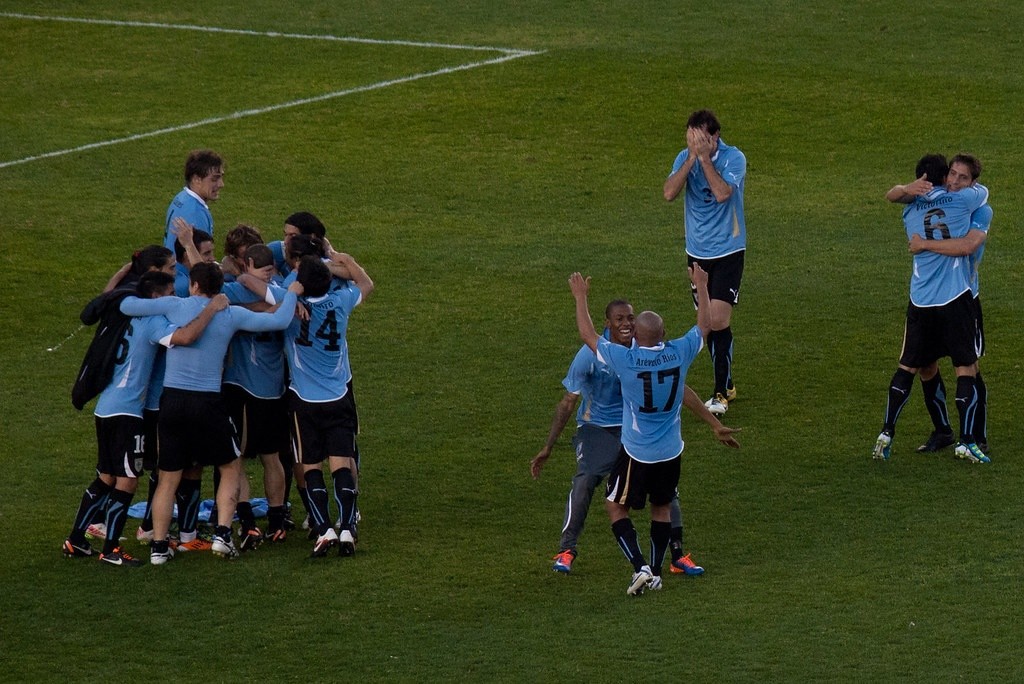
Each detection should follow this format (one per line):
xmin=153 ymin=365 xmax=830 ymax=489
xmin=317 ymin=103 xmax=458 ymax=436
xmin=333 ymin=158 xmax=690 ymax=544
xmin=285 ymin=211 xmax=327 ymax=241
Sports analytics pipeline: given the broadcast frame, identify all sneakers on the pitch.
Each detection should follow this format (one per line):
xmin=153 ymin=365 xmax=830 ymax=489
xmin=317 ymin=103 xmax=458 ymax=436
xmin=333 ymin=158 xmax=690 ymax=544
xmin=917 ymin=431 xmax=956 ymax=454
xmin=953 ymin=440 xmax=991 ymax=464
xmin=704 ymin=385 xmax=737 ymax=409
xmin=84 ymin=523 xmax=128 ymax=542
xmin=238 ymin=527 xmax=264 ymax=552
xmin=98 ymin=544 xmax=141 ymax=567
xmin=977 ymin=442 xmax=991 ymax=456
xmin=148 ymin=537 xmax=174 ymax=565
xmin=310 ymin=528 xmax=338 ymax=558
xmin=872 ymin=427 xmax=892 ymax=461
xmin=627 ymin=564 xmax=654 ymax=597
xmin=135 ymin=524 xmax=176 ymax=546
xmin=706 ymin=392 xmax=729 ymax=418
xmin=211 ymin=531 xmax=240 ymax=559
xmin=172 ymin=534 xmax=214 ymax=551
xmin=62 ymin=537 xmax=101 ymax=558
xmin=263 ymin=529 xmax=287 ymax=543
xmin=338 ymin=530 xmax=356 ymax=557
xmin=669 ymin=553 xmax=705 ymax=575
xmin=552 ymin=550 xmax=574 ymax=576
xmin=644 ymin=576 xmax=663 ymax=592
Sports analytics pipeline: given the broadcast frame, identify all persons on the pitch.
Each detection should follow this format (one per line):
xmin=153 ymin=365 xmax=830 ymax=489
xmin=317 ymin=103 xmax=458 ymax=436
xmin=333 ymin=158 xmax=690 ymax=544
xmin=60 ymin=212 xmax=375 ymax=567
xmin=872 ymin=152 xmax=993 ymax=464
xmin=530 ymin=299 xmax=742 ymax=576
xmin=162 ymin=149 xmax=225 ymax=261
xmin=569 ymin=262 xmax=713 ymax=596
xmin=663 ymin=109 xmax=747 ymax=417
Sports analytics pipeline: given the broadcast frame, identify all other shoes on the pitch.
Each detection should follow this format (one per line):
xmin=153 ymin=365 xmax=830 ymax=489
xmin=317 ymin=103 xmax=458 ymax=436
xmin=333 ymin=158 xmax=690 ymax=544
xmin=282 ymin=509 xmax=297 ymax=531
xmin=334 ymin=509 xmax=362 ymax=529
xmin=301 ymin=512 xmax=310 ymax=531
